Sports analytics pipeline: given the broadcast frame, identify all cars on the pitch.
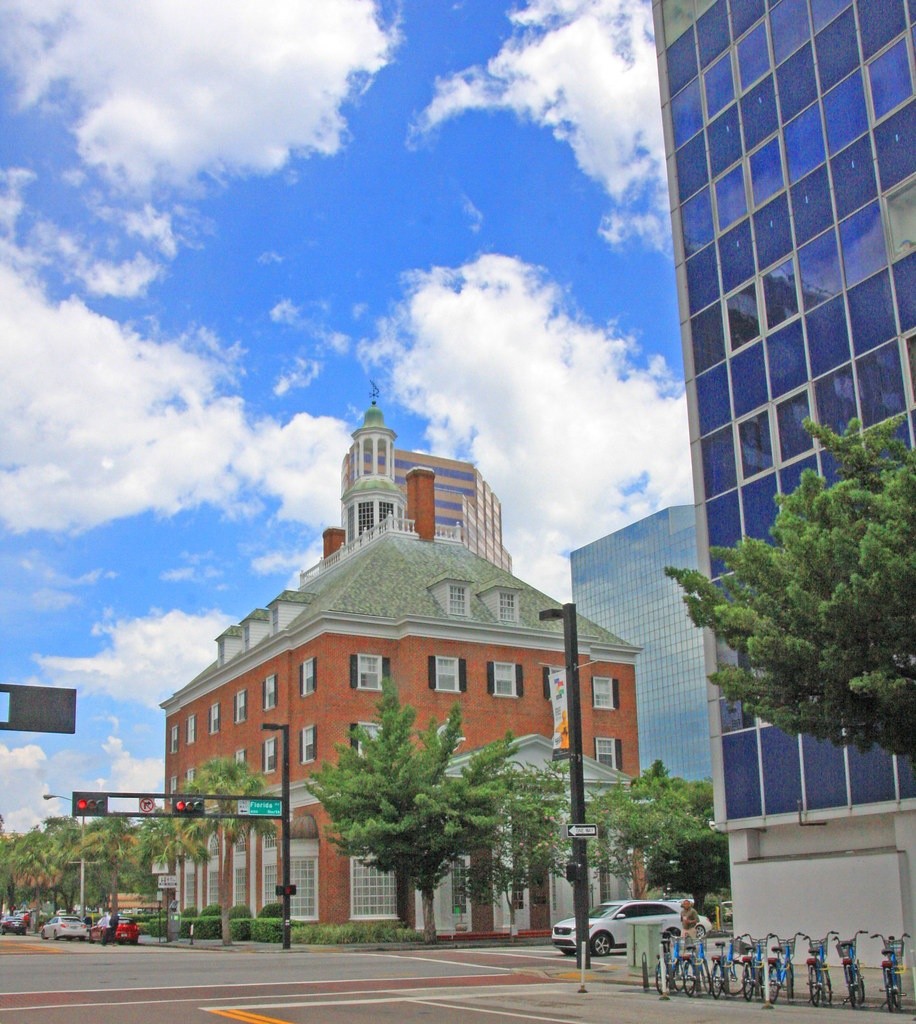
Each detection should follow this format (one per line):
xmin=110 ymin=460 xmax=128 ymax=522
xmin=721 ymin=901 xmax=733 ymax=921
xmin=0 ymin=916 xmax=27 ymax=936
xmin=551 ymin=898 xmax=714 ymax=957
xmin=90 ymin=916 xmax=140 ymax=945
xmin=11 ymin=904 xmax=146 ymax=926
xmin=40 ymin=916 xmax=88 ymax=941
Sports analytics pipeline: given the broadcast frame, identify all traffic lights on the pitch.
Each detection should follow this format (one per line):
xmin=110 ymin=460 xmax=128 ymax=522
xmin=73 ymin=795 xmax=108 ymax=814
xmin=285 ymin=886 xmax=292 ymax=894
xmin=172 ymin=797 xmax=205 ymax=816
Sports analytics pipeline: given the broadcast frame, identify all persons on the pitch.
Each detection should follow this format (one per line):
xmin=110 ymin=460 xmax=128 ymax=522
xmin=85 ymin=914 xmax=92 ymax=929
xmin=98 ymin=910 xmax=119 ymax=947
xmin=680 ymin=900 xmax=700 ymax=951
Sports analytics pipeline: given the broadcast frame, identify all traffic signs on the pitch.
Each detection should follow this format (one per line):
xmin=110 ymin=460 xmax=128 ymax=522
xmin=566 ymin=824 xmax=598 ymax=838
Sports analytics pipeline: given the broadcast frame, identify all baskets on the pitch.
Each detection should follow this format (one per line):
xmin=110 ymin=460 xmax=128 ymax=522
xmin=733 ymin=939 xmax=749 ymax=955
xmin=753 ymin=938 xmax=766 ymax=953
xmin=779 ymin=939 xmax=794 ymax=954
xmin=810 ymin=939 xmax=827 ymax=956
xmin=836 ymin=940 xmax=853 ymax=958
xmin=885 ymin=940 xmax=904 ymax=956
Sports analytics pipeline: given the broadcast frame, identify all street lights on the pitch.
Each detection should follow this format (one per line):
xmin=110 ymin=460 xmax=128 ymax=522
xmin=260 ymin=723 xmax=291 ymax=949
xmin=44 ymin=794 xmax=85 ymax=922
xmin=539 ymin=603 xmax=592 ymax=970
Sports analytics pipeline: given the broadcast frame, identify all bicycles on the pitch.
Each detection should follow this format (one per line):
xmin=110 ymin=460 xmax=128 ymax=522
xmin=739 ymin=933 xmax=775 ymax=1002
xmin=710 ymin=933 xmax=750 ymax=999
xmin=833 ymin=930 xmax=869 ymax=1009
xmin=681 ymin=932 xmax=711 ymax=998
xmin=656 ymin=930 xmax=691 ymax=995
xmin=767 ymin=932 xmax=806 ymax=1004
xmin=870 ymin=932 xmax=911 ymax=1013
xmin=803 ymin=931 xmax=840 ymax=1008
